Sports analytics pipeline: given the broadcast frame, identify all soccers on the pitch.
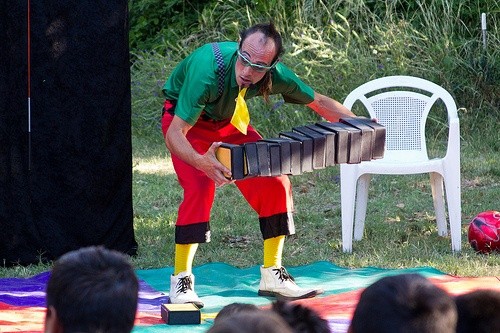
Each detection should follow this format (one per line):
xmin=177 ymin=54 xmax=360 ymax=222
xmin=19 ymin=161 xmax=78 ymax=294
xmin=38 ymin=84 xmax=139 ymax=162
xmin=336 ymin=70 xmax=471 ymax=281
xmin=468 ymin=211 xmax=500 ymax=255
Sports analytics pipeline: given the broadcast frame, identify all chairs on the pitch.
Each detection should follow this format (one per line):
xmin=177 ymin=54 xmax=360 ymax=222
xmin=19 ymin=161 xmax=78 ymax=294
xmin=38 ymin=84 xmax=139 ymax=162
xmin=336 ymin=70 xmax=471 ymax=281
xmin=339 ymin=75 xmax=461 ymax=253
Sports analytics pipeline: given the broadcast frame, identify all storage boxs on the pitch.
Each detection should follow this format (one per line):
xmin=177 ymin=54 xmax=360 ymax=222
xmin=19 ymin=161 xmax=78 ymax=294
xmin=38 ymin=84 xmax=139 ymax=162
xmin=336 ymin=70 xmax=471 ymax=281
xmin=210 ymin=117 xmax=386 ymax=181
xmin=161 ymin=303 xmax=201 ymax=325
